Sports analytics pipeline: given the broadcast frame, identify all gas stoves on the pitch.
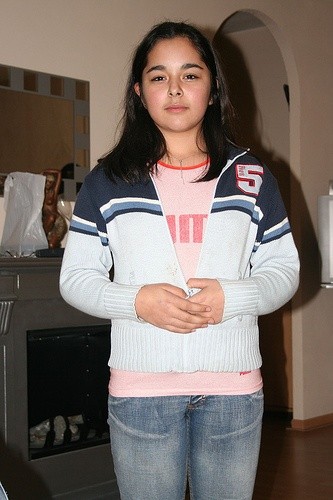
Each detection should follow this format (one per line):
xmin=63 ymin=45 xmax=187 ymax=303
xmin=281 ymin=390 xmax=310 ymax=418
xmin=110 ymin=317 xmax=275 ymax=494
xmin=0 ymin=249 xmax=64 ymax=267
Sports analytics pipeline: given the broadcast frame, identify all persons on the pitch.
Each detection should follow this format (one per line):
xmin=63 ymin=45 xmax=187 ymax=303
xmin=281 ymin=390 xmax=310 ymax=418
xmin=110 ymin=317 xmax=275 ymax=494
xmin=59 ymin=17 xmax=305 ymax=499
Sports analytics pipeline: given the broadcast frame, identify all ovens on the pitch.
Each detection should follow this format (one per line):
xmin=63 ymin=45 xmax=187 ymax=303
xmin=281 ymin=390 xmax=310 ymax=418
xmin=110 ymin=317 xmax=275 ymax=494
xmin=0 ymin=268 xmax=120 ymax=500
